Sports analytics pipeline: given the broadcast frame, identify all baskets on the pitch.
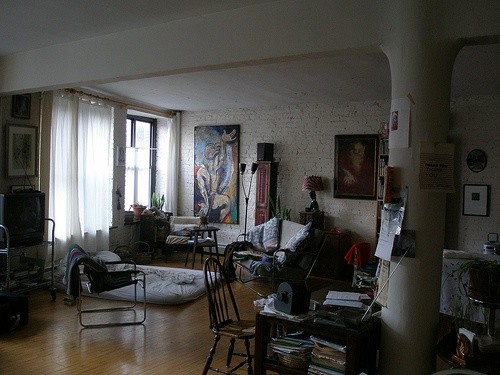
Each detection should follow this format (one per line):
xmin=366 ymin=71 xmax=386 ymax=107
xmin=277 ymin=353 xmax=309 ymax=369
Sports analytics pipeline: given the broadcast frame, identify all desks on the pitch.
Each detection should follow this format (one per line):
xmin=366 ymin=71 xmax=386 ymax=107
xmin=438 ymin=249 xmax=500 ymax=369
xmin=184 ymin=226 xmax=221 ymax=269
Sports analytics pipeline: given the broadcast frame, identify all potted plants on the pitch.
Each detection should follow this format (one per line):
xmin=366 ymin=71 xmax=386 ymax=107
xmin=130 ymin=204 xmax=147 ymax=220
xmin=444 ymin=257 xmax=500 ymax=333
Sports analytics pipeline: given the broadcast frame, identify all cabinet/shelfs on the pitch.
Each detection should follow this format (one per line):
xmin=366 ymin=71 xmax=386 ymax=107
xmin=254 ymin=304 xmax=381 ymax=375
xmin=299 ymin=211 xmax=324 ymax=230
xmin=309 ymin=230 xmax=350 ymax=281
xmin=140 ymin=212 xmax=173 ymax=254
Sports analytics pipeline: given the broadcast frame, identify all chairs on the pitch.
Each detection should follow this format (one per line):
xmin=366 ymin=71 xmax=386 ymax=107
xmin=344 ymin=241 xmax=377 ymax=289
xmin=161 ymin=215 xmax=208 ymax=263
xmin=62 ymin=243 xmax=147 ymax=328
xmin=201 ymin=257 xmax=255 ymax=375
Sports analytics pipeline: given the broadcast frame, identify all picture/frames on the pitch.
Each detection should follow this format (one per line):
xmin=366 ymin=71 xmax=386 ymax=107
xmin=333 ymin=134 xmax=379 ymax=200
xmin=11 ymin=93 xmax=32 ymax=119
xmin=5 ymin=123 xmax=40 ymax=179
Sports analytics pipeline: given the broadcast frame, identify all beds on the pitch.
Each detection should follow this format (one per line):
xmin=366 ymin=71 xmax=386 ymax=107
xmin=44 ymin=264 xmax=220 ymax=304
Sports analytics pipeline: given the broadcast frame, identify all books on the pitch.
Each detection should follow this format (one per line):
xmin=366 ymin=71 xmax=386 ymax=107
xmin=272 ymin=329 xmax=346 ymax=375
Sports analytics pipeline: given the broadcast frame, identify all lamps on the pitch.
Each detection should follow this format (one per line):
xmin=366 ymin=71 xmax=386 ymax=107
xmin=239 ymin=162 xmax=260 ymax=240
xmin=302 ymin=174 xmax=324 ymax=212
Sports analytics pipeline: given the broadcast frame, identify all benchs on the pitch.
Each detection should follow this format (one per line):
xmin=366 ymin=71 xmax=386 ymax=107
xmin=232 ymin=218 xmax=326 ymax=298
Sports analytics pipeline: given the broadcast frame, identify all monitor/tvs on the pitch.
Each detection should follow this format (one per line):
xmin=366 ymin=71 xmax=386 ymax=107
xmin=0 ymin=189 xmax=45 ymax=245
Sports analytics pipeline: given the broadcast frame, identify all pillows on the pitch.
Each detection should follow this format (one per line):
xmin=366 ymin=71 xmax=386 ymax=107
xmin=246 ymin=216 xmax=279 ymax=253
xmin=93 ymin=250 xmax=125 ymax=271
xmin=273 ymin=221 xmax=312 ymax=265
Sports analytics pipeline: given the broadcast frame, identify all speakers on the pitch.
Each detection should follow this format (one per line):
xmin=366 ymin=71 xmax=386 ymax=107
xmin=0 ymin=296 xmax=29 ymax=332
xmin=257 ymin=143 xmax=274 ymax=160
